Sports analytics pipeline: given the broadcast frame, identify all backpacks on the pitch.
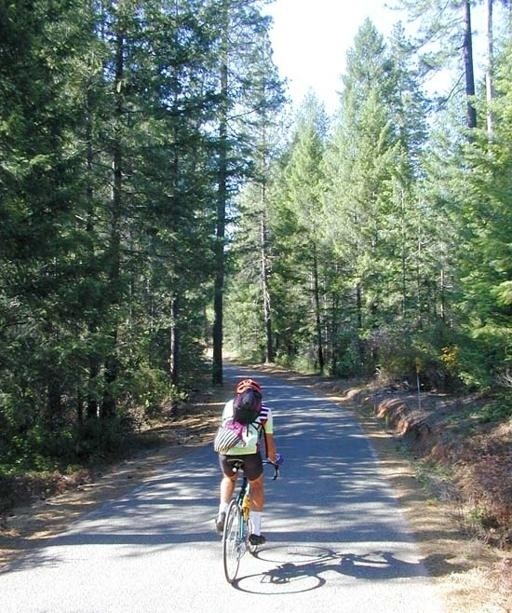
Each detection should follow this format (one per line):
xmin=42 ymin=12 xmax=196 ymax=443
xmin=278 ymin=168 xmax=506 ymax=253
xmin=233 ymin=389 xmax=261 ymax=426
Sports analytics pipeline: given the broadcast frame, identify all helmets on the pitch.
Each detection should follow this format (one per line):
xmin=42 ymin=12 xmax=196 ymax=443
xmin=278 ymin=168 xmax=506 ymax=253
xmin=234 ymin=379 xmax=261 ymax=394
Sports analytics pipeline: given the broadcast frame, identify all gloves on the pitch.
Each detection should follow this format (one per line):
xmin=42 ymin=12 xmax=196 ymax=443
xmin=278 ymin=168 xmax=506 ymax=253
xmin=272 ymin=452 xmax=284 ymax=465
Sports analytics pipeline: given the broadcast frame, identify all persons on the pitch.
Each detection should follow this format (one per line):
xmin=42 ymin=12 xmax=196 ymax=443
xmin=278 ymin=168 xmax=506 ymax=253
xmin=213 ymin=377 xmax=280 ymax=544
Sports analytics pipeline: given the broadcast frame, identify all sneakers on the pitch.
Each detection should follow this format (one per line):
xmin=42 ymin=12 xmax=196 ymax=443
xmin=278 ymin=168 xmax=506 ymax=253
xmin=248 ymin=534 xmax=266 ymax=545
xmin=215 ymin=512 xmax=226 ymax=530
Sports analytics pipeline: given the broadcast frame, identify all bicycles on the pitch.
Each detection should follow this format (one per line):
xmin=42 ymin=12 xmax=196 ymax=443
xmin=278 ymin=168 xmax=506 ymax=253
xmin=222 ymin=459 xmax=280 ymax=584
